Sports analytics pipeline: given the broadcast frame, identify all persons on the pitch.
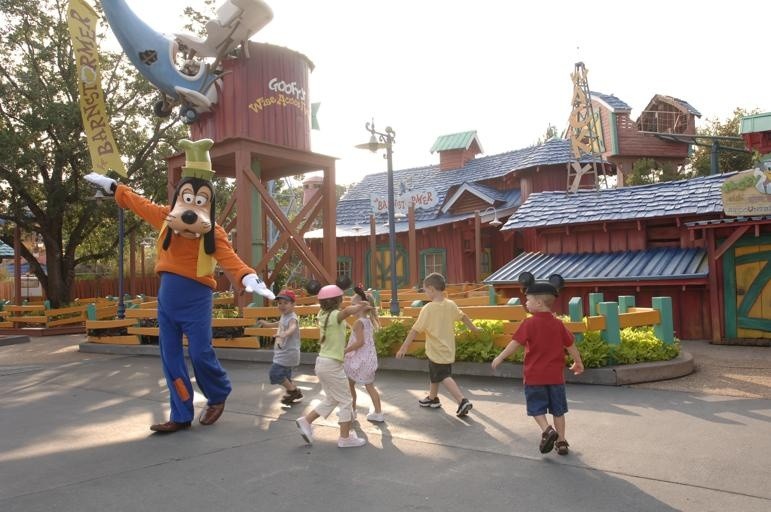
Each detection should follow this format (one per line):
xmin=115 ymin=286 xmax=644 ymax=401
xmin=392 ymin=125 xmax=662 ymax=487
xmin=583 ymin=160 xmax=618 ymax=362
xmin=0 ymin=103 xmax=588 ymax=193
xmin=296 ymin=284 xmax=373 ymax=447
xmin=335 ymin=281 xmax=384 ymax=422
xmin=395 ymin=271 xmax=481 ymax=417
xmin=491 ymin=272 xmax=584 ymax=454
xmin=260 ymin=289 xmax=303 ymax=406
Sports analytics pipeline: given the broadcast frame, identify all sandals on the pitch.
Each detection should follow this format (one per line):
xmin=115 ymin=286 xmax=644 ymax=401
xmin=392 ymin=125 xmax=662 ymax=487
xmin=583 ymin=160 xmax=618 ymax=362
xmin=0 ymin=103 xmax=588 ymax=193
xmin=539 ymin=426 xmax=559 ymax=454
xmin=556 ymin=438 xmax=570 ymax=455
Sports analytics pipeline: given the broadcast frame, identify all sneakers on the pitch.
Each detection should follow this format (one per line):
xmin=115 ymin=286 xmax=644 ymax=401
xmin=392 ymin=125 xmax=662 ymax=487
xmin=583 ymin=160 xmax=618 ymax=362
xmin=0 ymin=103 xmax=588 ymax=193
xmin=418 ymin=397 xmax=441 ymax=409
xmin=456 ymin=399 xmax=473 ymax=418
xmin=337 ymin=436 xmax=367 ymax=448
xmin=281 ymin=389 xmax=303 ymax=404
xmin=335 ymin=408 xmax=358 ymax=420
xmin=367 ymin=412 xmax=385 ymax=422
xmin=295 ymin=416 xmax=313 ymax=444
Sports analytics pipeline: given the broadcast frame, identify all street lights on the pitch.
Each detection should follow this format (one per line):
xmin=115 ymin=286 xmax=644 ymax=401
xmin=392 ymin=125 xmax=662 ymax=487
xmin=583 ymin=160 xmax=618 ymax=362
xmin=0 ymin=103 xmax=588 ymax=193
xmin=353 ymin=121 xmax=401 ymax=316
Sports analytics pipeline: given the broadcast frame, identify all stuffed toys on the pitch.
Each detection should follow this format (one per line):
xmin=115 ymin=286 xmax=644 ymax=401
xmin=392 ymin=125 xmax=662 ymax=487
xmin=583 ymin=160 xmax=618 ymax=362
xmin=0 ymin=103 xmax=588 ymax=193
xmin=83 ymin=138 xmax=277 ymax=432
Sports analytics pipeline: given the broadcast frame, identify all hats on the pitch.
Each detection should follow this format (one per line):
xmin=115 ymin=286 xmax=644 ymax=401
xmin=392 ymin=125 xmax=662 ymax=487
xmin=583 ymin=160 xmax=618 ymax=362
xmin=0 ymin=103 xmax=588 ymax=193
xmin=517 ymin=267 xmax=566 ymax=299
xmin=275 ymin=289 xmax=296 ymax=302
xmin=306 ymin=274 xmax=352 ymax=299
